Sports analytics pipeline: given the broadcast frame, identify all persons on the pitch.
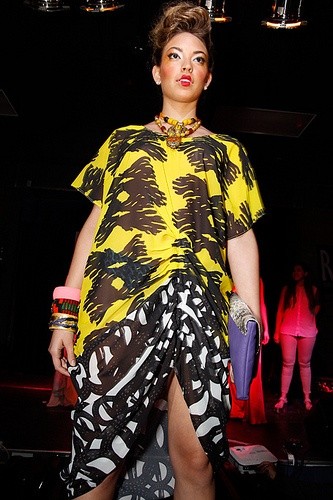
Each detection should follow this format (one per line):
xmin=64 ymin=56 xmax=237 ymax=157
xmin=228 ymin=260 xmax=319 ymax=500
xmin=45 ymin=333 xmax=78 ymax=406
xmin=42 ymin=3 xmax=266 ymax=500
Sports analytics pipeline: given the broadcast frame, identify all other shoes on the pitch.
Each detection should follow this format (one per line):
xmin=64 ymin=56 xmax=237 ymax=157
xmin=304 ymin=398 xmax=313 ymax=411
xmin=273 ymin=398 xmax=287 ymax=409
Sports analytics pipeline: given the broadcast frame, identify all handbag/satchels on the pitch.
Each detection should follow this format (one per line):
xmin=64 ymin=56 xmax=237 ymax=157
xmin=228 ymin=292 xmax=260 ymax=400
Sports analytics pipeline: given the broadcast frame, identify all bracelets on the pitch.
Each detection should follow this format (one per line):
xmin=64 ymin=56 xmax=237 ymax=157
xmin=48 ymin=287 xmax=81 ymax=332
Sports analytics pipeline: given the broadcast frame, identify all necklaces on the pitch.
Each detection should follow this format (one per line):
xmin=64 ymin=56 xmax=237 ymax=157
xmin=155 ymin=112 xmax=201 ymax=149
